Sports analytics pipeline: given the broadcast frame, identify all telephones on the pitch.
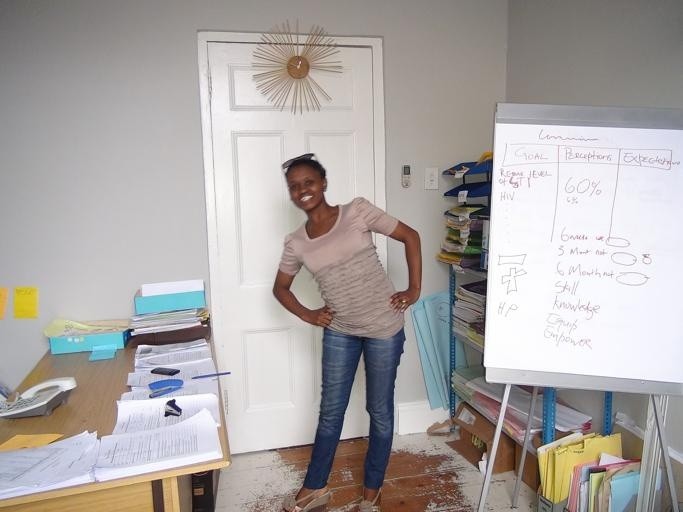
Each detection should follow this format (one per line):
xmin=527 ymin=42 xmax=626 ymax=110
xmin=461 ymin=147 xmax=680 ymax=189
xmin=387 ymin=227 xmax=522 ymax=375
xmin=0 ymin=377 xmax=78 ymax=418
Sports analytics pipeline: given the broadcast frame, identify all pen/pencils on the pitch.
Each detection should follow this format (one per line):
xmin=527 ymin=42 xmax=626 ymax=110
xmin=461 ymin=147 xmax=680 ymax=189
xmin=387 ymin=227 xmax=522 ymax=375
xmin=192 ymin=372 xmax=230 ymax=379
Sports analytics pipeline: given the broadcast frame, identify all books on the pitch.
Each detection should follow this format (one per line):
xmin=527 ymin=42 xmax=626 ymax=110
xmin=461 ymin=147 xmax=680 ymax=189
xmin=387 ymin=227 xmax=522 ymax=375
xmin=450 ymin=365 xmax=642 ymax=512
xmin=127 ymin=308 xmax=202 ymax=337
xmin=450 ymin=278 xmax=486 ymax=351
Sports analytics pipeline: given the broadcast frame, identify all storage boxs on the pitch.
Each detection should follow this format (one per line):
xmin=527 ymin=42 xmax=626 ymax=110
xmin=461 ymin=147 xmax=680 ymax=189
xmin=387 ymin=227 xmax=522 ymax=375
xmin=444 ymin=400 xmax=575 ymax=492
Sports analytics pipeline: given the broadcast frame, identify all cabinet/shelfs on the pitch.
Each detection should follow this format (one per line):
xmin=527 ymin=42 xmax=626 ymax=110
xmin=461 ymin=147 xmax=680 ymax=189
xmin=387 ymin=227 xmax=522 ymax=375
xmin=442 ymin=160 xmax=612 ymax=446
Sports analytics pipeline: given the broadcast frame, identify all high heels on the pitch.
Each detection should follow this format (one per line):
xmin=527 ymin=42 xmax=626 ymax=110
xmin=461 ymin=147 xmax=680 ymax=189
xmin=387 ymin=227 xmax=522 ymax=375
xmin=279 ymin=488 xmax=330 ymax=512
xmin=359 ymin=487 xmax=382 ymax=512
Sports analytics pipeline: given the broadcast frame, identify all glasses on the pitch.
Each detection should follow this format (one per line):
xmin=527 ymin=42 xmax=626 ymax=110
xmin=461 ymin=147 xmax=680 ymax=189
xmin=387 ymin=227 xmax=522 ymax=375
xmin=282 ymin=154 xmax=314 ymax=172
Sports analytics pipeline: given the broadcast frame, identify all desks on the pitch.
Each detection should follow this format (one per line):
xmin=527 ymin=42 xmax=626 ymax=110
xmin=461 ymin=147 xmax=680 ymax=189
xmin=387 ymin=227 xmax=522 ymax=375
xmin=0 ymin=327 xmax=231 ymax=512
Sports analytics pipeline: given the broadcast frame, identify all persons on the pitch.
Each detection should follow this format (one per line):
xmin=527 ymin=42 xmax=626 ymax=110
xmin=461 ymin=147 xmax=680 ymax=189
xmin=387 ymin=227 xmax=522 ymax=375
xmin=272 ymin=158 xmax=423 ymax=512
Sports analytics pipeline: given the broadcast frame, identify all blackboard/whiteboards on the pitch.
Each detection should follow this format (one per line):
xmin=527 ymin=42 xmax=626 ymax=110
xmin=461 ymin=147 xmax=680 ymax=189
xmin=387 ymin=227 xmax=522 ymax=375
xmin=482 ymin=104 xmax=683 ymax=393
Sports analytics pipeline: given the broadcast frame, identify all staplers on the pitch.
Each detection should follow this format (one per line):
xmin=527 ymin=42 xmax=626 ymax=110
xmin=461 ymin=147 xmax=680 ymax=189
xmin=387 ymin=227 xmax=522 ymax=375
xmin=149 ymin=380 xmax=183 ymax=398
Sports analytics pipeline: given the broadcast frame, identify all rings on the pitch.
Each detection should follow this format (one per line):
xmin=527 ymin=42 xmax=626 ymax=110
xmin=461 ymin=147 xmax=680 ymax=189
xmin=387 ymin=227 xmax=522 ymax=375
xmin=400 ymin=299 xmax=408 ymax=303
